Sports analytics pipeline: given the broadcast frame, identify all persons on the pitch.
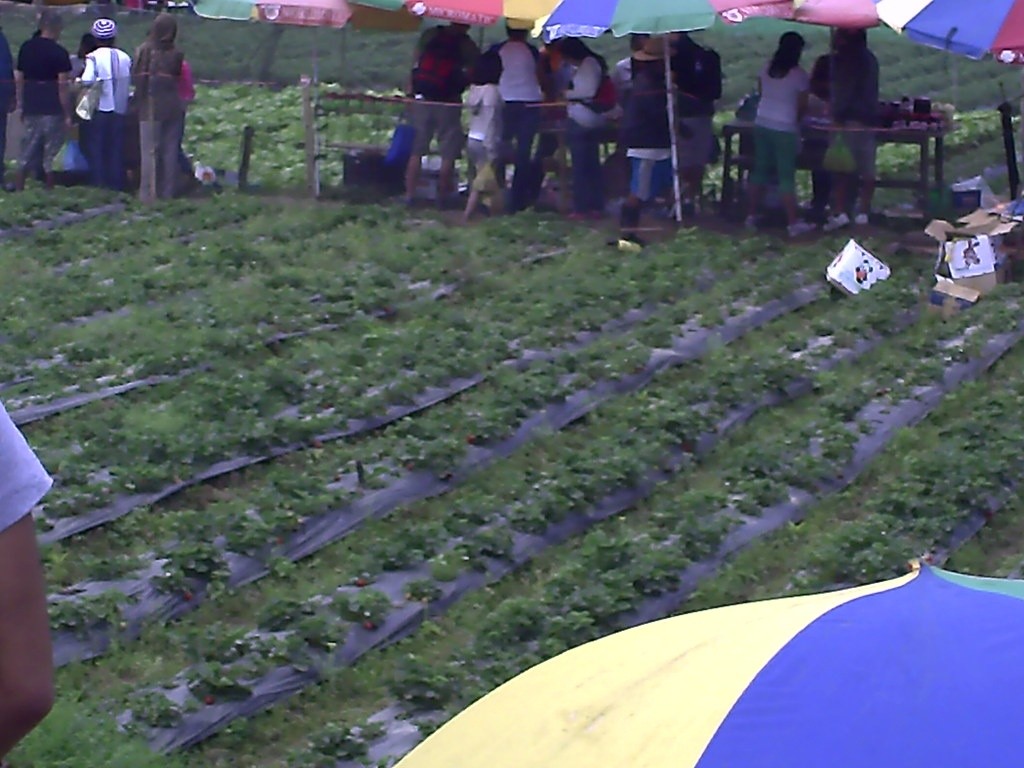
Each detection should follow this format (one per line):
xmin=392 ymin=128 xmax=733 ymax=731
xmin=745 ymin=31 xmax=817 ymax=238
xmin=70 ymin=18 xmax=132 ymax=191
xmin=0 ymin=32 xmax=17 ymax=191
xmin=15 ymin=10 xmax=73 ymax=192
xmin=805 ymin=23 xmax=881 ymax=233
xmin=402 ymin=22 xmax=723 ymax=244
xmin=132 ymin=13 xmax=187 ymax=198
xmin=0 ymin=401 xmax=55 ymax=759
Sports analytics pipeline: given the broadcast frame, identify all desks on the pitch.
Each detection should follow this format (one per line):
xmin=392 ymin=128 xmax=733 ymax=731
xmin=718 ymin=120 xmax=957 ymax=232
xmin=537 ymin=118 xmax=710 ymax=219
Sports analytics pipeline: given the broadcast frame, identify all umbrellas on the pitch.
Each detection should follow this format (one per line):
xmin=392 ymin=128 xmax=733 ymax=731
xmin=531 ymin=0 xmax=797 ymax=221
xmin=709 ymin=0 xmax=883 ymax=29
xmin=875 ymin=1 xmax=1024 ymax=67
xmin=195 ymin=0 xmax=423 ymax=197
xmin=398 ymin=558 xmax=1024 ymax=768
xmin=352 ymin=0 xmax=563 ymax=43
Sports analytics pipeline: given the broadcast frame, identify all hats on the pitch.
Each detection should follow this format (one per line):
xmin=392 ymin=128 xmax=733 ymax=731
xmin=93 ymin=18 xmax=117 ymax=39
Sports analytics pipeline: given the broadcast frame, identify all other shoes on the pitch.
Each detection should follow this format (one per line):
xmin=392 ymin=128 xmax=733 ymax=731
xmin=787 ymin=221 xmax=817 ymax=238
xmin=744 ymin=217 xmax=755 ymax=232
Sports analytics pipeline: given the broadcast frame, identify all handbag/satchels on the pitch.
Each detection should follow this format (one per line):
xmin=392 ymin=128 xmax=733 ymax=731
xmin=588 ymin=74 xmax=617 ymax=114
xmin=74 ymin=54 xmax=103 ymax=123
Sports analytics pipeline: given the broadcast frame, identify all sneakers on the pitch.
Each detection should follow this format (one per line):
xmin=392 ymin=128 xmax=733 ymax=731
xmin=823 ymin=214 xmax=849 ymax=234
xmin=854 ymin=214 xmax=867 ymax=225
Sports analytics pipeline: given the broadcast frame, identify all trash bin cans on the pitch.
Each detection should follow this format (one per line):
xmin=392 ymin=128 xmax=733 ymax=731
xmin=344 ymin=151 xmax=381 ymax=197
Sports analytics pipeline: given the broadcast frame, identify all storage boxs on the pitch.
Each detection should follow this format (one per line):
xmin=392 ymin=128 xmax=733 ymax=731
xmin=344 ymin=149 xmax=460 ymax=200
xmin=824 ymin=211 xmax=1018 ymax=308
xmin=951 ymin=188 xmax=980 ymax=209
xmin=624 ymin=148 xmax=674 ymax=200
